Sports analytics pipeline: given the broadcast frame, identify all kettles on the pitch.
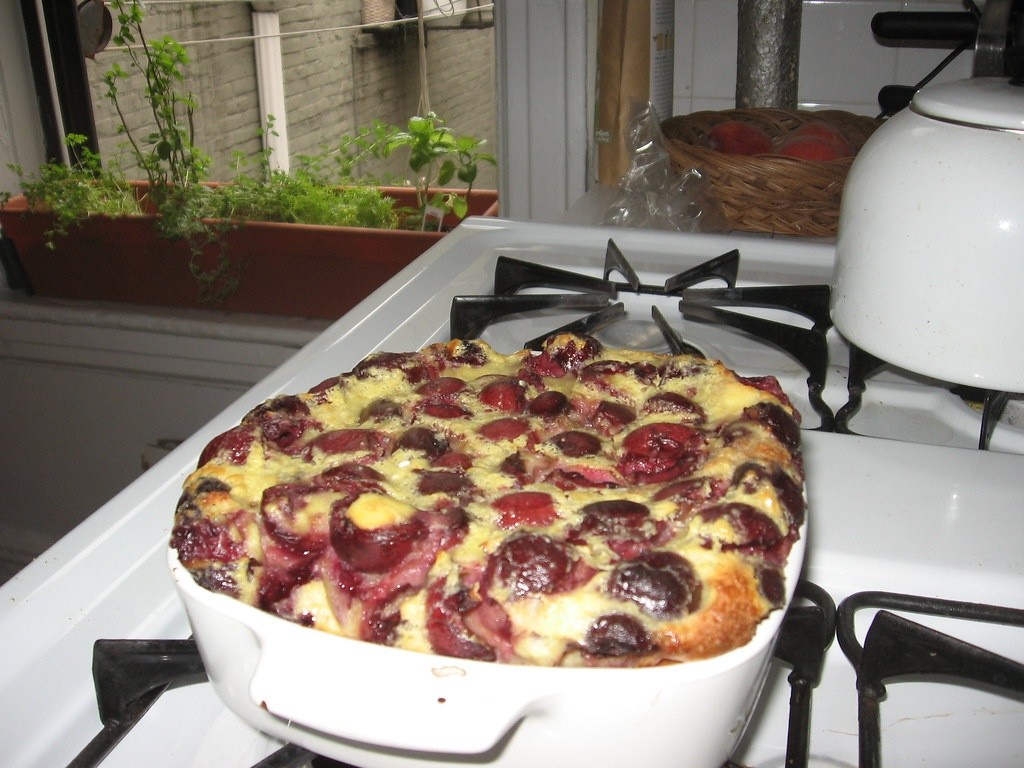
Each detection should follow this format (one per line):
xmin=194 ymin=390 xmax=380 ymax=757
xmin=829 ymin=7 xmax=1024 ymax=394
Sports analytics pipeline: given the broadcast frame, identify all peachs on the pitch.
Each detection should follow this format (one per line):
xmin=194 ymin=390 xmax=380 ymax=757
xmin=700 ymin=118 xmax=852 ymax=163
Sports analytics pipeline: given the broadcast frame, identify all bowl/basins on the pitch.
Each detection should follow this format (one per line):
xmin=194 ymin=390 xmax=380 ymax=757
xmin=168 ymin=481 xmax=809 ymax=768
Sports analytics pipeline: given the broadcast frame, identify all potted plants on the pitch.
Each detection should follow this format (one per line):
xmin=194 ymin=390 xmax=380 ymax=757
xmin=0 ymin=0 xmax=499 ymax=322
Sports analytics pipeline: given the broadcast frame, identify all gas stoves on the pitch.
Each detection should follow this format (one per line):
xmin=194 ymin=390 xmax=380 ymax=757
xmin=0 ymin=213 xmax=1024 ymax=768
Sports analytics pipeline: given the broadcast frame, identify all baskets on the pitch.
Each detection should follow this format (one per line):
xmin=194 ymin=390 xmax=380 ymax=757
xmin=660 ymin=106 xmax=888 ymax=239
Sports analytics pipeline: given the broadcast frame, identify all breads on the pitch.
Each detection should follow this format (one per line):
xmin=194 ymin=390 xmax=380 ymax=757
xmin=170 ymin=327 xmax=808 ymax=669
